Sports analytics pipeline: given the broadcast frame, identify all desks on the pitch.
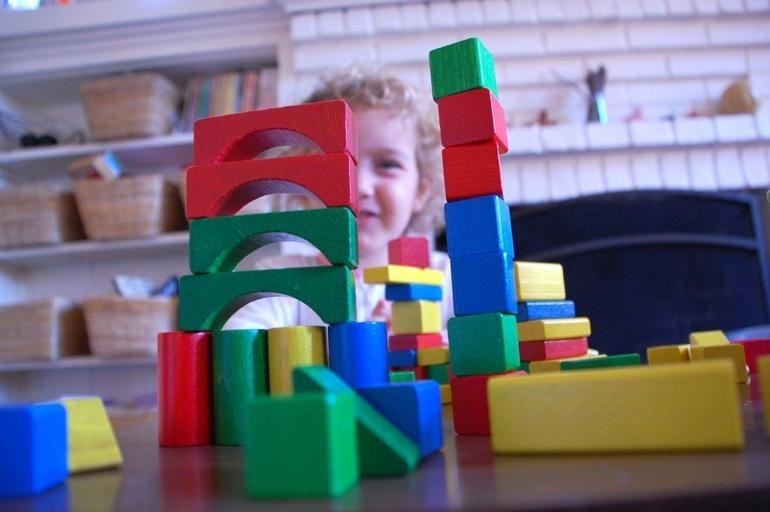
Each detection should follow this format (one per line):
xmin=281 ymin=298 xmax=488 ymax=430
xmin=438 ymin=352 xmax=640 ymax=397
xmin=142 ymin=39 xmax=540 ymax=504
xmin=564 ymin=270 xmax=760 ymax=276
xmin=1 ymin=406 xmax=770 ymax=511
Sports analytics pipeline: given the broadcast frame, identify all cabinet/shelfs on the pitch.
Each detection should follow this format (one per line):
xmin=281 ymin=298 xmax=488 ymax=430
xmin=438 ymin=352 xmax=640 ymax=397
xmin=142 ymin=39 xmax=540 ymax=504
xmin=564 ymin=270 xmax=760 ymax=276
xmin=1 ymin=11 xmax=291 ymax=374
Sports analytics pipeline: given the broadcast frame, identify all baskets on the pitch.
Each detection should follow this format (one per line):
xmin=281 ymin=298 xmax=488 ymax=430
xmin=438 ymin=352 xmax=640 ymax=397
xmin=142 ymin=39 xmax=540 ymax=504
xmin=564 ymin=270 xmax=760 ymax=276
xmin=68 ymin=176 xmax=178 ymax=242
xmin=0 ymin=181 xmax=79 ymax=245
xmin=76 ymin=72 xmax=181 ymax=141
xmin=79 ymin=295 xmax=178 ymax=359
xmin=0 ymin=298 xmax=88 ymax=362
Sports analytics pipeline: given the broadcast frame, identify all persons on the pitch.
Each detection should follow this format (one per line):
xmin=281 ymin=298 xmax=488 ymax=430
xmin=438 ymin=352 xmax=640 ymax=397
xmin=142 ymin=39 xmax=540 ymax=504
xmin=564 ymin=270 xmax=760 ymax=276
xmin=219 ymin=68 xmax=453 ymax=348
